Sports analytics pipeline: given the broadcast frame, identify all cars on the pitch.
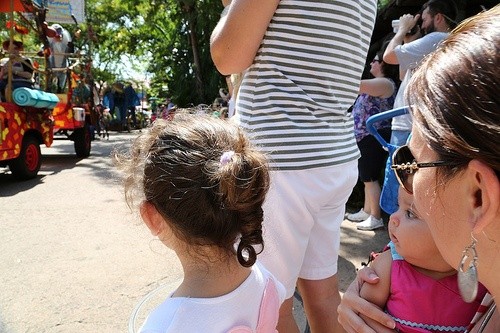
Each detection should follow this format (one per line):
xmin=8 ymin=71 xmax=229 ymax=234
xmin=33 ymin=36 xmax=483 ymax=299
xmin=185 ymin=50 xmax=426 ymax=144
xmin=0 ymin=0 xmax=93 ymax=179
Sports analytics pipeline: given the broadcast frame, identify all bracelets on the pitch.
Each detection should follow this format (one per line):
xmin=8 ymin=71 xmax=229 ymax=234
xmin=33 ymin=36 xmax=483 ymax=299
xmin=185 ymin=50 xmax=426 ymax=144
xmin=220 ymin=4 xmax=230 ymax=15
xmin=15 ymin=70 xmax=18 ymax=75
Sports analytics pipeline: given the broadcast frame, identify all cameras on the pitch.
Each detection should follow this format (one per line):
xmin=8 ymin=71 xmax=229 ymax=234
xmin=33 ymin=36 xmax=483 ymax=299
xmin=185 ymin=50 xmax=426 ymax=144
xmin=392 ymin=20 xmax=417 ymax=35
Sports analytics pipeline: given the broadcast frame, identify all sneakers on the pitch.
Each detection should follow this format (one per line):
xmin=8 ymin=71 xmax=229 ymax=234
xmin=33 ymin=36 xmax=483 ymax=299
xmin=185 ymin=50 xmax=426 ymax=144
xmin=357 ymin=215 xmax=384 ymax=230
xmin=348 ymin=208 xmax=371 ymax=221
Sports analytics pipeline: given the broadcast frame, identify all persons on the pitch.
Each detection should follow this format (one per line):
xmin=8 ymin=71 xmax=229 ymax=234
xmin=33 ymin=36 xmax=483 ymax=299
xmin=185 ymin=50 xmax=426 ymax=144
xmin=359 ymin=173 xmax=487 ymax=333
xmin=337 ymin=4 xmax=500 ymax=333
xmin=114 ymin=108 xmax=286 ymax=333
xmin=0 ymin=0 xmax=457 ymax=231
xmin=207 ymin=0 xmax=378 ymax=333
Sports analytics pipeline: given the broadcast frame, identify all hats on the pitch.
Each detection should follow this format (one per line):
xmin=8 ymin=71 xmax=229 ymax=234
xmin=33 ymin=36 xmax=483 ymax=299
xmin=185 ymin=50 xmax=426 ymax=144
xmin=160 ymin=105 xmax=166 ymax=108
xmin=3 ymin=35 xmax=23 ymax=50
xmin=47 ymin=24 xmax=63 ymax=37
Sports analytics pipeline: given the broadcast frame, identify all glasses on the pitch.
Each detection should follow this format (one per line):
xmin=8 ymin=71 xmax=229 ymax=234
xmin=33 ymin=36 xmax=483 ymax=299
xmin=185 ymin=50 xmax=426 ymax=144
xmin=372 ymin=58 xmax=379 ymax=63
xmin=390 ymin=144 xmax=447 ymax=195
